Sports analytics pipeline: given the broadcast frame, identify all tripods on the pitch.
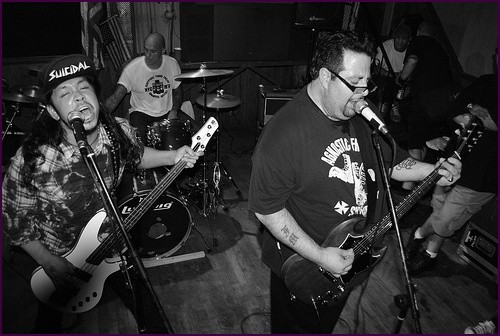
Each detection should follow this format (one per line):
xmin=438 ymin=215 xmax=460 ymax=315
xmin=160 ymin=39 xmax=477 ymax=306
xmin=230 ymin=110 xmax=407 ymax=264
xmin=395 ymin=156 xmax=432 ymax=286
xmin=174 ymin=77 xmax=244 ymax=246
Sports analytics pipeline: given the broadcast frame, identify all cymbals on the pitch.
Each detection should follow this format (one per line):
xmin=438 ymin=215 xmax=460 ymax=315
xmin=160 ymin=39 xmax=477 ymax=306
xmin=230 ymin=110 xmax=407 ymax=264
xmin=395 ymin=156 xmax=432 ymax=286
xmin=196 ymin=92 xmax=242 ymax=108
xmin=0 ymin=92 xmax=43 ymax=104
xmin=23 ymin=86 xmax=42 ymax=100
xmin=174 ymin=68 xmax=235 ymax=81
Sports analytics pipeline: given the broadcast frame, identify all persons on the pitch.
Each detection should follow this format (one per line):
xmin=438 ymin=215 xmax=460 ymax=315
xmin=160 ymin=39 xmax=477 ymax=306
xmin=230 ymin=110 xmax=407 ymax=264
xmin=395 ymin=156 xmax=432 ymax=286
xmin=401 ymin=48 xmax=498 ymax=277
xmin=464 ymin=320 xmax=496 ymax=334
xmin=104 ymin=32 xmax=202 ymax=147
xmin=247 ymin=22 xmax=462 ymax=334
xmin=373 ymin=22 xmax=420 ymax=121
xmin=2 ymin=54 xmax=204 ymax=334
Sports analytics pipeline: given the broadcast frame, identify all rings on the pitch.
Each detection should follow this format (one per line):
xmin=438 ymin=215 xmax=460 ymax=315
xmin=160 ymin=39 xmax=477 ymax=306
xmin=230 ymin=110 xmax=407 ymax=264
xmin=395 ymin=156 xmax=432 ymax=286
xmin=448 ymin=177 xmax=453 ymax=182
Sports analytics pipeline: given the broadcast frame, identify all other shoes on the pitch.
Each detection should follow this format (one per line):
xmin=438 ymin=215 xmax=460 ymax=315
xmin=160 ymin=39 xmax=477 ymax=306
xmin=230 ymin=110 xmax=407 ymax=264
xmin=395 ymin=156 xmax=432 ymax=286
xmin=404 ymin=227 xmax=424 ymax=259
xmin=377 ymin=100 xmax=401 ymax=123
xmin=418 ymin=248 xmax=439 ymax=271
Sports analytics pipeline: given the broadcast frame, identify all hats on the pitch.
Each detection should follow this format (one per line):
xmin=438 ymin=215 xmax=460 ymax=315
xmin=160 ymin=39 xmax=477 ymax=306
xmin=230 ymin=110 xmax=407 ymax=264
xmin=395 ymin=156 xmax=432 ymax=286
xmin=37 ymin=53 xmax=99 ymax=104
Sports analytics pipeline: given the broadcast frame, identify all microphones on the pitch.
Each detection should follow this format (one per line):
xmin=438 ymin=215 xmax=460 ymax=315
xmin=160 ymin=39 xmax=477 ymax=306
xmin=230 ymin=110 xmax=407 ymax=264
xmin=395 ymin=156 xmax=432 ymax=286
xmin=354 ymin=98 xmax=392 ymax=138
xmin=67 ymin=110 xmax=87 ymax=157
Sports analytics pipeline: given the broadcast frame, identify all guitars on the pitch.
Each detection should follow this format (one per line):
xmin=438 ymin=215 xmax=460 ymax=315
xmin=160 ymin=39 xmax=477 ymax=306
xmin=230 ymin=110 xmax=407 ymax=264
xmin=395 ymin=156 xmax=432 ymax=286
xmin=29 ymin=115 xmax=219 ymax=314
xmin=279 ymin=114 xmax=486 ymax=322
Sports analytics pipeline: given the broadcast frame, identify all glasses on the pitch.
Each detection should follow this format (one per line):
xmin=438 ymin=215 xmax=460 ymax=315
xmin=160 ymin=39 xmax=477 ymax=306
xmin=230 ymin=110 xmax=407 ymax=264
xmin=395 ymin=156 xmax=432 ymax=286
xmin=327 ymin=67 xmax=378 ymax=94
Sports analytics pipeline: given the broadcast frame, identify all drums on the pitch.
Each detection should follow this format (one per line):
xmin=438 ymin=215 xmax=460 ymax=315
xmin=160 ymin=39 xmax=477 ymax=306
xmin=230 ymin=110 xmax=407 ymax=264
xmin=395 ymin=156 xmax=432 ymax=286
xmin=145 ymin=116 xmax=201 ymax=161
xmin=113 ymin=168 xmax=194 ymax=263
